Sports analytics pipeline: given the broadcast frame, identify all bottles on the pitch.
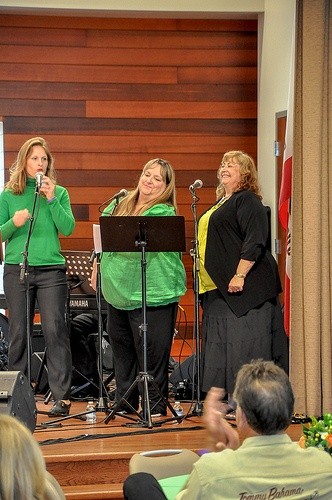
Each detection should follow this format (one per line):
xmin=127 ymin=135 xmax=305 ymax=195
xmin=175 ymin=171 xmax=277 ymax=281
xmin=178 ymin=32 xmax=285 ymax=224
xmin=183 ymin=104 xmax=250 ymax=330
xmin=177 ymin=381 xmax=186 ymax=399
xmin=173 ymin=402 xmax=183 ymax=422
xmin=85 ymin=401 xmax=96 ymax=422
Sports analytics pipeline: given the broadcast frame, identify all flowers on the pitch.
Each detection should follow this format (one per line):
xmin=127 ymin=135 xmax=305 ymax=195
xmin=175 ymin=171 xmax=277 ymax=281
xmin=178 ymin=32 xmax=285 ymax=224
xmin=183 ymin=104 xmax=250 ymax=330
xmin=298 ymin=414 xmax=332 ymax=455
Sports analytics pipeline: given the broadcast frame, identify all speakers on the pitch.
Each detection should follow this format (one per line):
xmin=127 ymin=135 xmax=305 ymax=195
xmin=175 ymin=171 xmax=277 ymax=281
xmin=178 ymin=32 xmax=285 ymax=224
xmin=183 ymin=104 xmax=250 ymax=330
xmin=0 ymin=371 xmax=37 ymax=434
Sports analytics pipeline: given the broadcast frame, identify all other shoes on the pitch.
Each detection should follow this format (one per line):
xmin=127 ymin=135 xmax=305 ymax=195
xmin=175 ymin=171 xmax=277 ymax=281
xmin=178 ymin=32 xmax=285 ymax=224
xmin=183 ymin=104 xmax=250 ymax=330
xmin=107 ymin=404 xmax=130 ymax=413
xmin=150 ymin=408 xmax=164 ymax=417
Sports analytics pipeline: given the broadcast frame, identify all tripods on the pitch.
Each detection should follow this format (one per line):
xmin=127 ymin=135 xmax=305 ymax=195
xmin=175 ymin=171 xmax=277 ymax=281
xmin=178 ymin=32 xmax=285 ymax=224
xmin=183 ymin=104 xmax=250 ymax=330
xmin=41 ymin=193 xmax=237 ymax=430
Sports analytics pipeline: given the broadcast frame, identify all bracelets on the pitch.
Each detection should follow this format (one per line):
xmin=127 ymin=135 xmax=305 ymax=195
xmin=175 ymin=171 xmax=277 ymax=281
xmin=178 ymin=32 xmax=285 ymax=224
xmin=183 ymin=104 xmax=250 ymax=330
xmin=234 ymin=273 xmax=245 ymax=279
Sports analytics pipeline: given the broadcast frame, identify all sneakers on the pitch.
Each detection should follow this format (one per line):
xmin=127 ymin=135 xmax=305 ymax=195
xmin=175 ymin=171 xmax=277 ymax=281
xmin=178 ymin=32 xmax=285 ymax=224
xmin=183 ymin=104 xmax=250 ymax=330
xmin=49 ymin=399 xmax=71 ymax=417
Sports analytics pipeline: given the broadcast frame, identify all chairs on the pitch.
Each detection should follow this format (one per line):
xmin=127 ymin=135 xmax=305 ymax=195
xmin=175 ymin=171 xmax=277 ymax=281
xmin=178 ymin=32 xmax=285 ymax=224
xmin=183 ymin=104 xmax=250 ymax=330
xmin=128 ymin=449 xmax=200 ymax=500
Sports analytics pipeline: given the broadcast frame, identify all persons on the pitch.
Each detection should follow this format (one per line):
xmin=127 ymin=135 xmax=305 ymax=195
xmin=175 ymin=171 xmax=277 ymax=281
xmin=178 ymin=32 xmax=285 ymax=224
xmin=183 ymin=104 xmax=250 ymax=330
xmin=0 ymin=136 xmax=73 ymax=416
xmin=69 ymin=312 xmax=100 ymax=401
xmin=123 ymin=358 xmax=332 ymax=499
xmin=0 ymin=415 xmax=65 ymax=500
xmin=91 ymin=158 xmax=186 ymax=417
xmin=193 ymin=150 xmax=289 ymax=420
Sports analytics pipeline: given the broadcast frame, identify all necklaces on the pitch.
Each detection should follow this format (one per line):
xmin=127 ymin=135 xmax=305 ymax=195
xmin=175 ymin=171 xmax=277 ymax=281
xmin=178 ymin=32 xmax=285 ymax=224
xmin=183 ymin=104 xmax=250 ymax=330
xmin=215 ymin=192 xmax=234 ymax=205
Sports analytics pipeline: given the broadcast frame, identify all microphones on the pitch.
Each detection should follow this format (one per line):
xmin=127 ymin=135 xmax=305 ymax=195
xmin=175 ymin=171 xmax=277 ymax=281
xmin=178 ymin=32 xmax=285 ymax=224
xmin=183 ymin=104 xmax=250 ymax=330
xmin=114 ymin=189 xmax=127 ymax=199
xmin=35 ymin=172 xmax=44 ymax=187
xmin=189 ymin=180 xmax=203 ymax=190
